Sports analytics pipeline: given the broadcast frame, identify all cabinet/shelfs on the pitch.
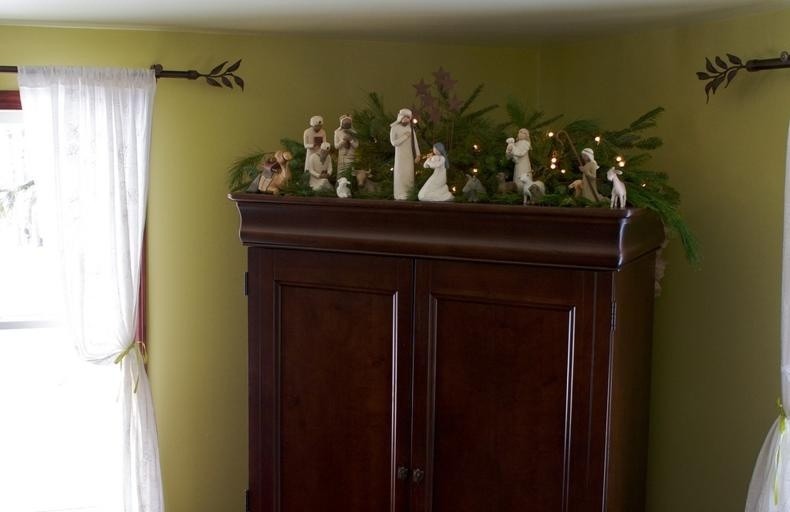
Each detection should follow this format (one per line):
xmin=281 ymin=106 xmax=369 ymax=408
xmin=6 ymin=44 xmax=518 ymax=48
xmin=227 ymin=187 xmax=664 ymax=512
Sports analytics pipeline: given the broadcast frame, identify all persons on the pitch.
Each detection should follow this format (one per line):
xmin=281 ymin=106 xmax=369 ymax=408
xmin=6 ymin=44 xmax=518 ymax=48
xmin=506 ymin=128 xmax=532 ymax=191
xmin=244 ymin=106 xmax=628 ymax=211
xmin=303 ymin=114 xmax=359 ymax=191
xmin=248 ymin=151 xmax=292 ymax=195
xmin=417 ymin=142 xmax=456 ymax=201
xmin=579 ymin=148 xmax=603 ymax=202
xmin=389 ymin=109 xmax=421 ymax=200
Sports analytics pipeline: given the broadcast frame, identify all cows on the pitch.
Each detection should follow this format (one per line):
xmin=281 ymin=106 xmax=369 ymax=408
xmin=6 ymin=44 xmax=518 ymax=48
xmin=350 ymin=167 xmax=381 ymax=193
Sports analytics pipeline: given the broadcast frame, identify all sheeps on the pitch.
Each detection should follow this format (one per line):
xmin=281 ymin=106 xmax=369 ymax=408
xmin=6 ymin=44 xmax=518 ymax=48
xmin=495 ymin=173 xmax=517 ymax=194
xmin=607 ymin=167 xmax=627 ymax=209
xmin=519 ymin=174 xmax=546 ymax=204
xmin=336 ymin=177 xmax=352 ymax=198
